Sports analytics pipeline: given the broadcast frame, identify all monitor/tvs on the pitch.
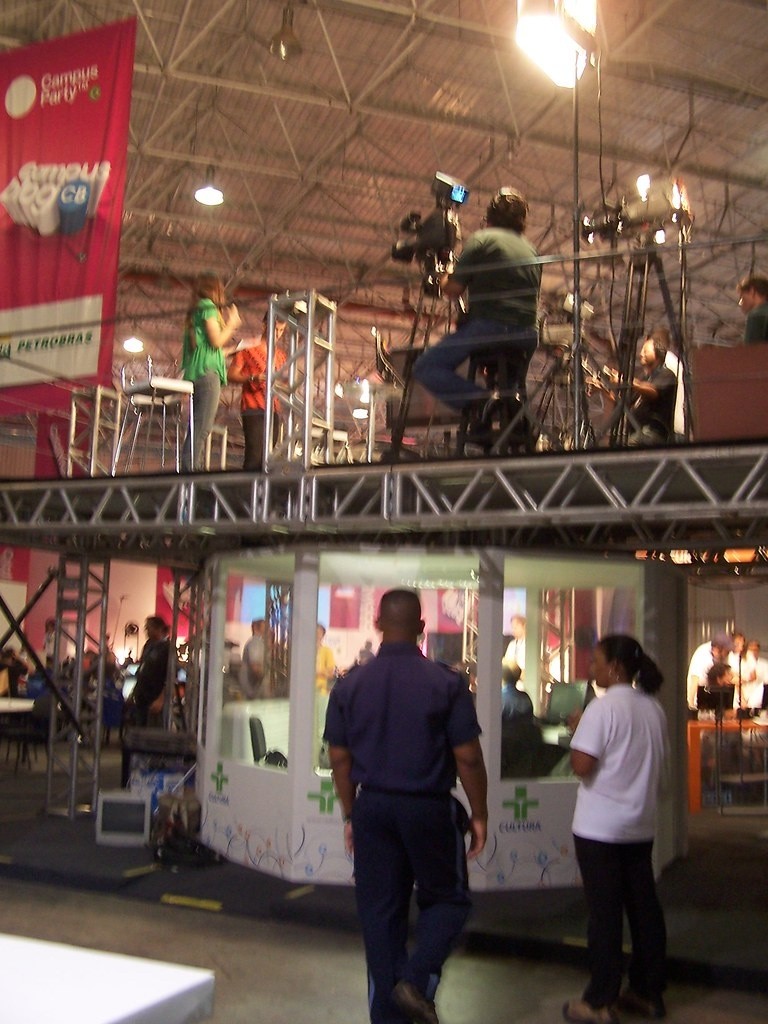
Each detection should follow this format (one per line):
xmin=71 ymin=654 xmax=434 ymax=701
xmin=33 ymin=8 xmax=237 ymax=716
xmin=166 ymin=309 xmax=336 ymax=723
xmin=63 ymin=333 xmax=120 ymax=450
xmin=97 ymin=789 xmax=151 ymax=846
xmin=547 ymin=682 xmax=596 ymax=725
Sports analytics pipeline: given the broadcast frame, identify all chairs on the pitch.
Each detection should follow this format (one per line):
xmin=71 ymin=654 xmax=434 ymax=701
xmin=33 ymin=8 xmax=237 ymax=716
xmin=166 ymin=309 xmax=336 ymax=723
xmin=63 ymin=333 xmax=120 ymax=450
xmin=249 ymin=716 xmax=267 ymax=763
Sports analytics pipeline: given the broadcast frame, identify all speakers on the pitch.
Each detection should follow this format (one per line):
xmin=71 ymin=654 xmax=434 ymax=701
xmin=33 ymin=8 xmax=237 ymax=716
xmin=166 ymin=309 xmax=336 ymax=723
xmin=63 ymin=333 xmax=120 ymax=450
xmin=0 ymin=416 xmax=37 ymax=481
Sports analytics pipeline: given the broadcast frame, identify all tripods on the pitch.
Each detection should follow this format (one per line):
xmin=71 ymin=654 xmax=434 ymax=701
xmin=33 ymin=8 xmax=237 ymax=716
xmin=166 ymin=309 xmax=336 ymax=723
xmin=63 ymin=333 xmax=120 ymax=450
xmin=383 ymin=231 xmax=694 ymax=465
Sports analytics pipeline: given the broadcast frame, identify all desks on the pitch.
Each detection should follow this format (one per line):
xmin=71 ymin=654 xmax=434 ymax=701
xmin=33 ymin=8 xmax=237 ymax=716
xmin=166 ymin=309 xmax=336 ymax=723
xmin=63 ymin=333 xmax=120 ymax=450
xmin=688 ymin=720 xmax=768 ymax=818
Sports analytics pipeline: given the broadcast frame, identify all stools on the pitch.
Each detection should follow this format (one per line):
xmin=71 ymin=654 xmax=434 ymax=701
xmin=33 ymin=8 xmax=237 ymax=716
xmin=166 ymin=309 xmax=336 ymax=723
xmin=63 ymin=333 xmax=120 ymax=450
xmin=63 ymin=355 xmax=227 ymax=480
xmin=454 ymin=345 xmax=531 ymax=457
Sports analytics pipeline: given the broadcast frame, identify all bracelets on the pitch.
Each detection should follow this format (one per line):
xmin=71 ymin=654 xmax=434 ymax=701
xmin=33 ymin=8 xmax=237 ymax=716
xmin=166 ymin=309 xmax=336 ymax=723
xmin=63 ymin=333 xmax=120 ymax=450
xmin=343 ymin=815 xmax=352 ymax=823
xmin=250 ymin=375 xmax=254 ymax=382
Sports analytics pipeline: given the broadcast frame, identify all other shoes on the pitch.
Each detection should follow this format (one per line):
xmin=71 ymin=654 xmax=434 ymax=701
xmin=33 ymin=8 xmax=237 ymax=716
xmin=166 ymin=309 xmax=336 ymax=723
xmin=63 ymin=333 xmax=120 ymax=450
xmin=615 ymin=986 xmax=666 ymax=1018
xmin=468 ymin=391 xmax=502 ymax=433
xmin=563 ymin=1000 xmax=618 ymax=1024
xmin=392 ymin=980 xmax=438 ymax=1024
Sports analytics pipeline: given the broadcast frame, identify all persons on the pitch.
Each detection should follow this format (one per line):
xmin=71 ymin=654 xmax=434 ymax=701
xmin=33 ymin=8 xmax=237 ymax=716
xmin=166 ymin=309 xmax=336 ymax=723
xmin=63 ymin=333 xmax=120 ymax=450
xmin=222 ymin=619 xmax=282 ymax=707
xmin=737 ymin=274 xmax=768 ymax=345
xmin=0 ymin=616 xmax=186 ymax=729
xmin=685 ymin=630 xmax=768 ymax=771
xmin=452 ymin=615 xmax=545 ymax=778
xmin=608 ymin=336 xmax=678 ymax=448
xmin=315 ymin=626 xmax=336 ymax=693
xmin=183 ymin=269 xmax=240 ymax=471
xmin=415 ymin=185 xmax=547 ymax=452
xmin=357 ymin=641 xmax=374 ymax=665
xmin=561 ymin=632 xmax=669 ymax=1024
xmin=228 ymin=311 xmax=288 ymax=473
xmin=322 ymin=589 xmax=487 ymax=1024
